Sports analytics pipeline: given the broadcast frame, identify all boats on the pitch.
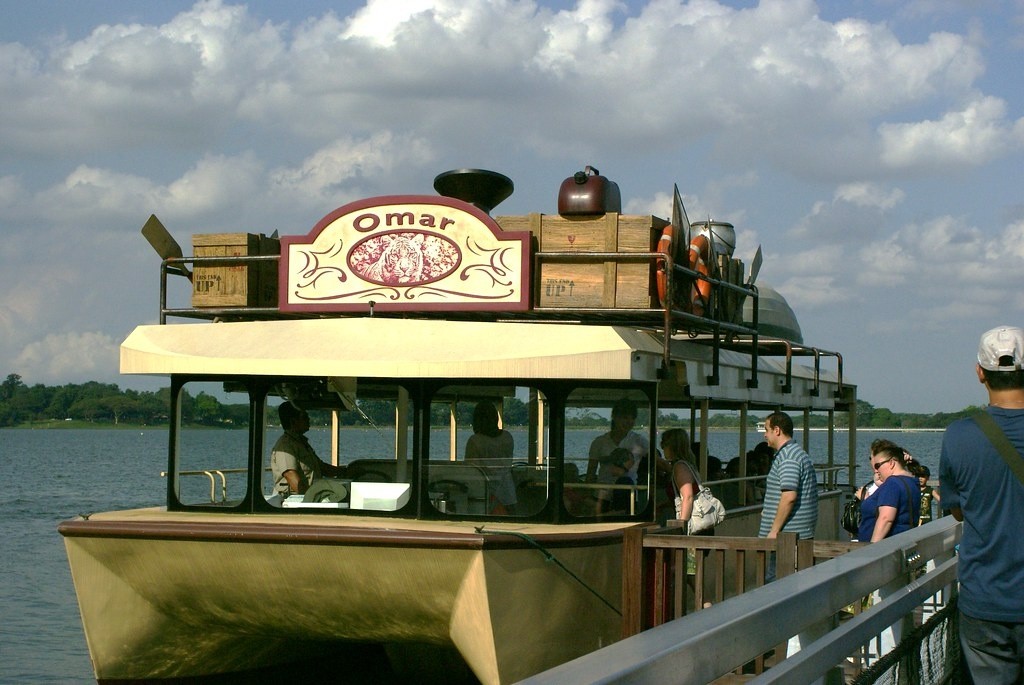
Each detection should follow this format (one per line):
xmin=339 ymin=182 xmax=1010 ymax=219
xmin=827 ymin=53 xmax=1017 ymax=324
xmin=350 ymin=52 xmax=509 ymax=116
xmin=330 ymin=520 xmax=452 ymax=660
xmin=57 ymin=168 xmax=859 ymax=685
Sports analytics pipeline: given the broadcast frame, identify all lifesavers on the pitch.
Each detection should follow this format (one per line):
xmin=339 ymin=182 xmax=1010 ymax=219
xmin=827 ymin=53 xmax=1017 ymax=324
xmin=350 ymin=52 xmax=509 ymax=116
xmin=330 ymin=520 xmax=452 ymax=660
xmin=687 ymin=234 xmax=712 ymax=316
xmin=655 ymin=226 xmax=676 ymax=308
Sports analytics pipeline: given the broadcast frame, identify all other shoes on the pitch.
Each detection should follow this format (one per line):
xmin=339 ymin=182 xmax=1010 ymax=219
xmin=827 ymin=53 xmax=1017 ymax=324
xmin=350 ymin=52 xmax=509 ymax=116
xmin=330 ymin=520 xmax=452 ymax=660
xmin=846 ymin=656 xmax=865 ymax=664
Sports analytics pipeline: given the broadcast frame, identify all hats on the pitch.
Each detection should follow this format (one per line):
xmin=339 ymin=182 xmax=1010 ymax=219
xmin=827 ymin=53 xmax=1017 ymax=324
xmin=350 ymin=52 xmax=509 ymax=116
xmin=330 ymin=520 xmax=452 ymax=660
xmin=302 ymin=480 xmax=347 ymax=502
xmin=599 ymin=448 xmax=634 ymax=471
xmin=918 ymin=466 xmax=930 ymax=477
xmin=977 ymin=326 xmax=1024 ymax=373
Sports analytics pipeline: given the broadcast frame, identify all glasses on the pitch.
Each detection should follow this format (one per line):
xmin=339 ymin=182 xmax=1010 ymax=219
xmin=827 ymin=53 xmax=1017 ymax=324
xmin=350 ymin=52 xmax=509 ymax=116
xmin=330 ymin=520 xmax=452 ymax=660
xmin=875 ymin=458 xmax=897 ymax=469
xmin=661 ymin=440 xmax=670 ymax=448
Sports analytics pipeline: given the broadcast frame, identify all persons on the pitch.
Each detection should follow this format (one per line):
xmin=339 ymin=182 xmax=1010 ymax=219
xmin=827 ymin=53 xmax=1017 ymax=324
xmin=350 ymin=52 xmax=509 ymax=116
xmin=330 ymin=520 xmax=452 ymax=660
xmin=758 ymin=412 xmax=818 ymax=584
xmin=841 ymin=439 xmax=941 ymax=617
xmin=270 ymin=401 xmax=367 ymax=502
xmin=563 ymin=398 xmax=775 ymax=609
xmin=463 ymin=400 xmax=518 ymax=516
xmin=938 ymin=326 xmax=1024 ymax=685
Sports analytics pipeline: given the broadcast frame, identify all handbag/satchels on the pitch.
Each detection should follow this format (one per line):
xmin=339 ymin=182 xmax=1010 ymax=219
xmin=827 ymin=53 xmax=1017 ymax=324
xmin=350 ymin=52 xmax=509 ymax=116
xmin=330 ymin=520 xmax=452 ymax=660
xmin=671 ymin=460 xmax=725 ymax=536
xmin=841 ymin=496 xmax=863 ymax=534
